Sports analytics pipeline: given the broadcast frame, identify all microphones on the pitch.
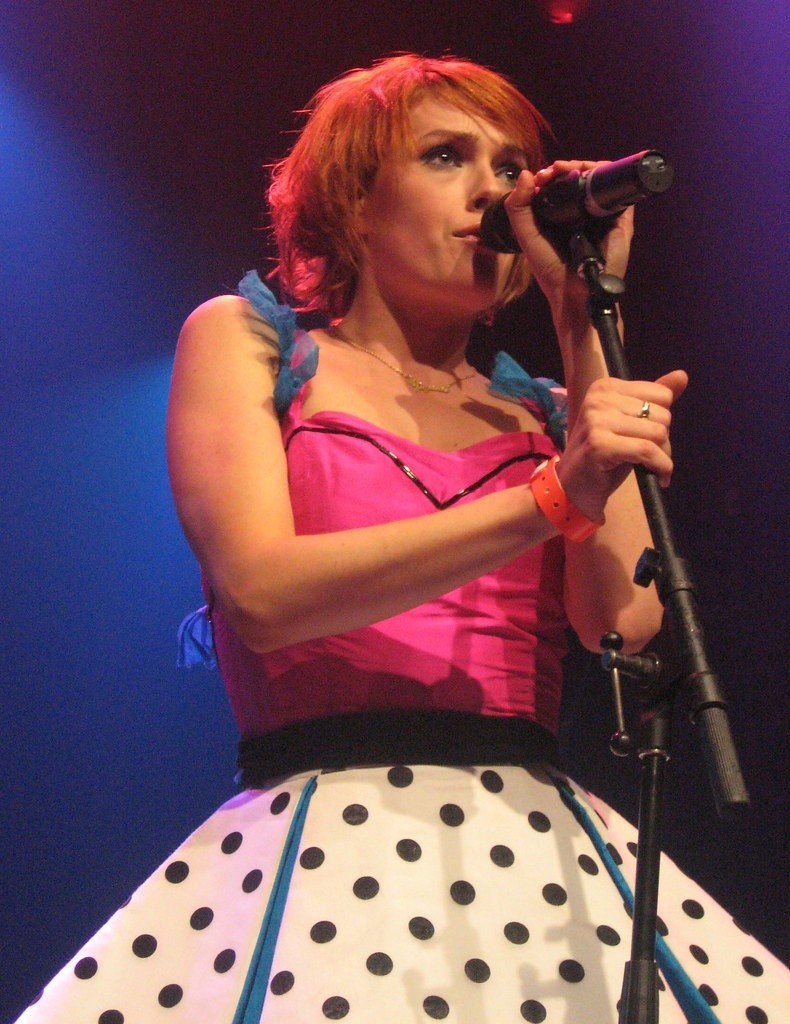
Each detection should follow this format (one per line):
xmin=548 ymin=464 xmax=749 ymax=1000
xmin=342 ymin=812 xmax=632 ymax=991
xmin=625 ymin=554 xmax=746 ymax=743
xmin=481 ymin=150 xmax=674 ymax=255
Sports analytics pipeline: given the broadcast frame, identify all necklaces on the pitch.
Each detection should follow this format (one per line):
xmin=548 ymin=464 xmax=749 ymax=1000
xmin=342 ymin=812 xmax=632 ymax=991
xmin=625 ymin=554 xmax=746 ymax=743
xmin=326 ymin=324 xmax=477 ymax=392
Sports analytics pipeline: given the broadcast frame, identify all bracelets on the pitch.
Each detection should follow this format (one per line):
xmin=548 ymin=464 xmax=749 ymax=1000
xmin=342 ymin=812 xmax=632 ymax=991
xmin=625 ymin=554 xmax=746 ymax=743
xmin=531 ymin=453 xmax=607 ymax=545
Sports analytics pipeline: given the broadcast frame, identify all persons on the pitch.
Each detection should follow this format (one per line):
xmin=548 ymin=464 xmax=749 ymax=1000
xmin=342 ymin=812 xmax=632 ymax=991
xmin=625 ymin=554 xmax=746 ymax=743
xmin=15 ymin=49 xmax=790 ymax=1024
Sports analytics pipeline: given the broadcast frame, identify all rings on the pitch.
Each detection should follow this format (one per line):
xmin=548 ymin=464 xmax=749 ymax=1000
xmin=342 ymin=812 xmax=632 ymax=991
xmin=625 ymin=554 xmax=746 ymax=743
xmin=636 ymin=399 xmax=651 ymax=418
